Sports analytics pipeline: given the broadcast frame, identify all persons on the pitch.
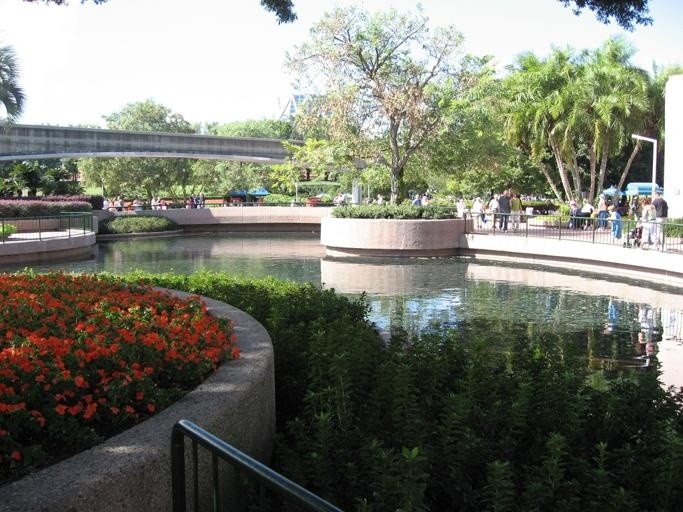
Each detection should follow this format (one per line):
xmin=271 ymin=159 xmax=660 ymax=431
xmin=603 ymin=297 xmax=664 ymax=360
xmin=102 ymin=192 xmax=204 ymax=211
